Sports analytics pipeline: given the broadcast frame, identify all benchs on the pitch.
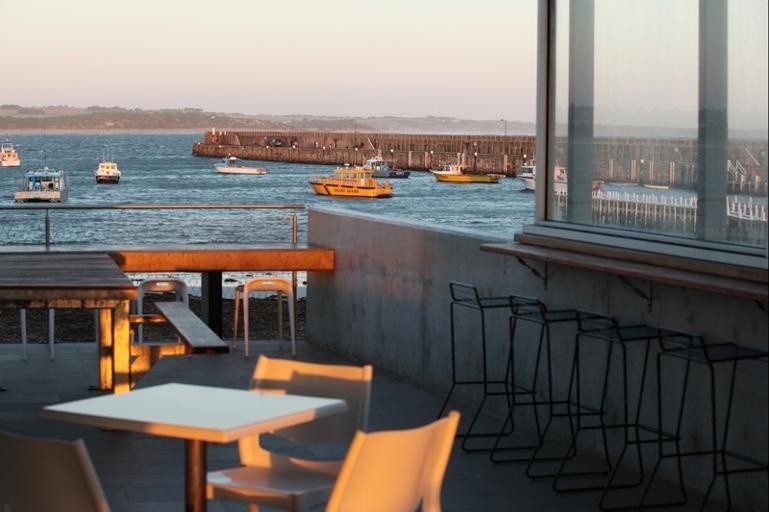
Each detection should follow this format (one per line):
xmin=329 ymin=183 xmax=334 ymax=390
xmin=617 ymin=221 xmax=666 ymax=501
xmin=146 ymin=299 xmax=229 ymax=358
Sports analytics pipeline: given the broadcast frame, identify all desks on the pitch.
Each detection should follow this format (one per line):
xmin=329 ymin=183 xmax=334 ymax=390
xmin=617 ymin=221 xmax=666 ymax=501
xmin=0 ymin=250 xmax=140 ymax=397
xmin=44 ymin=381 xmax=348 ymax=512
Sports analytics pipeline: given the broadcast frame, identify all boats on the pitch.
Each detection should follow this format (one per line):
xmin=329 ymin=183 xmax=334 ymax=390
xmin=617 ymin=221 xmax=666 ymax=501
xmin=214 ymin=153 xmax=268 ymax=176
xmin=357 ymin=157 xmax=410 ymax=180
xmin=0 ymin=138 xmax=21 ymax=168
xmin=309 ymin=165 xmax=392 ymax=201
xmin=519 ymin=163 xmax=605 ymax=200
xmin=13 ymin=170 xmax=66 ymax=204
xmin=431 ymin=163 xmax=504 ymax=183
xmin=95 ymin=160 xmax=121 ymax=183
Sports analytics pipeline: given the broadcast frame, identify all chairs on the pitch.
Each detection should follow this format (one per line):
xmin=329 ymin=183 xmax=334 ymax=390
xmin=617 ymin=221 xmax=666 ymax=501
xmin=231 ymin=276 xmax=301 ymax=358
xmin=637 ymin=325 xmax=768 ymax=511
xmin=326 ymin=408 xmax=461 ymax=512
xmin=205 ymin=351 xmax=374 ymax=512
xmin=489 ymin=292 xmax=623 ymax=478
xmin=434 ymin=280 xmax=547 ymax=459
xmin=1 ymin=428 xmax=114 ymax=512
xmin=137 ymin=277 xmax=190 ymax=347
xmin=554 ymin=307 xmax=694 ymax=511
